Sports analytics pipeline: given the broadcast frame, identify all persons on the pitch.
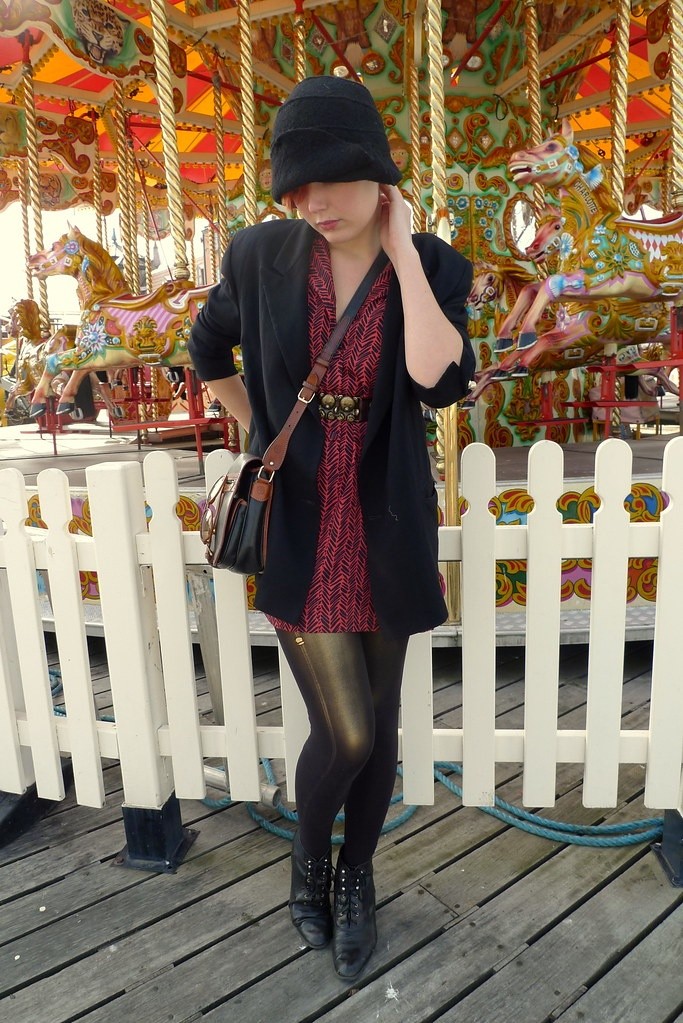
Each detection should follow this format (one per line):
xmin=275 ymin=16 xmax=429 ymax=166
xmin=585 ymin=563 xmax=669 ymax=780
xmin=187 ymin=76 xmax=476 ymax=982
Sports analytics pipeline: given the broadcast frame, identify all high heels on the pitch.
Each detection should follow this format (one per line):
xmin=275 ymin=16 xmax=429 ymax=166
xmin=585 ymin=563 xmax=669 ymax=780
xmin=333 ymin=857 xmax=379 ymax=980
xmin=289 ymin=827 xmax=335 ymax=950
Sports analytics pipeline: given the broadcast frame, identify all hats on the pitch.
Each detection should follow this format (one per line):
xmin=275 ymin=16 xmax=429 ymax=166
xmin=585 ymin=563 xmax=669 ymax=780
xmin=270 ymin=76 xmax=403 ymax=205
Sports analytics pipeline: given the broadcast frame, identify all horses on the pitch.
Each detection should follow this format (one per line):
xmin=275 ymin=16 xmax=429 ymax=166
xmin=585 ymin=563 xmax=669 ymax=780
xmin=460 ymin=117 xmax=683 ymax=410
xmin=0 ymin=299 xmax=125 ymax=420
xmin=27 ymin=219 xmax=245 ymax=418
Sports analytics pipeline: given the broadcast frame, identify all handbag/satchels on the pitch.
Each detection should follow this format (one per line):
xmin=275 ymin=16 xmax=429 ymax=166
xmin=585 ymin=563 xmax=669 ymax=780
xmin=199 ymin=246 xmax=389 ymax=574
